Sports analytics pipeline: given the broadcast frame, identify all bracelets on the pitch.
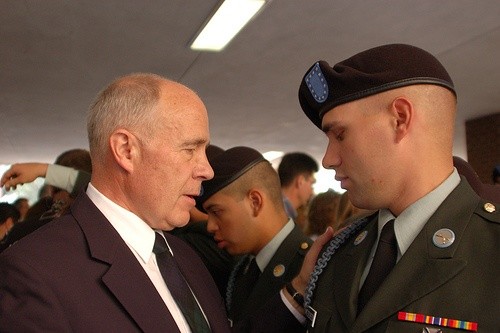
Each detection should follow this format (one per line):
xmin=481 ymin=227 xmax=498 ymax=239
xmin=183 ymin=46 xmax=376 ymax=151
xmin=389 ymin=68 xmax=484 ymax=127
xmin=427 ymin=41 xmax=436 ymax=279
xmin=286 ymin=282 xmax=305 ymax=309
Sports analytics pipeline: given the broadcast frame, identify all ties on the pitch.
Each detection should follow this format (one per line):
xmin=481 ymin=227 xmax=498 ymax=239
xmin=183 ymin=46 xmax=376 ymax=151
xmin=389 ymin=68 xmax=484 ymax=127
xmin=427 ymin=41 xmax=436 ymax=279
xmin=357 ymin=220 xmax=399 ymax=312
xmin=244 ymin=259 xmax=261 ymax=296
xmin=153 ymin=232 xmax=210 ymax=333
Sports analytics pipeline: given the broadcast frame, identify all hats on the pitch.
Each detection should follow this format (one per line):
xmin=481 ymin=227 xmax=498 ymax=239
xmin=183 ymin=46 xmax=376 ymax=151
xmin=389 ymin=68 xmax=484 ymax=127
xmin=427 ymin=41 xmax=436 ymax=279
xmin=194 ymin=146 xmax=266 ymax=214
xmin=298 ymin=43 xmax=457 ymax=129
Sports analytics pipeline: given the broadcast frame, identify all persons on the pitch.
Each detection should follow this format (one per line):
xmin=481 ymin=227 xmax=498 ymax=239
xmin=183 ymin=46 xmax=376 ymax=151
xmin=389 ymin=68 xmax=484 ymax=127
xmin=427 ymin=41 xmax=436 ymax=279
xmin=0 ymin=149 xmax=92 ymax=253
xmin=278 ymin=153 xmax=368 ymax=243
xmin=298 ymin=44 xmax=500 ymax=333
xmin=0 ymin=73 xmax=334 ymax=333
xmin=0 ymin=146 xmax=313 ymax=333
